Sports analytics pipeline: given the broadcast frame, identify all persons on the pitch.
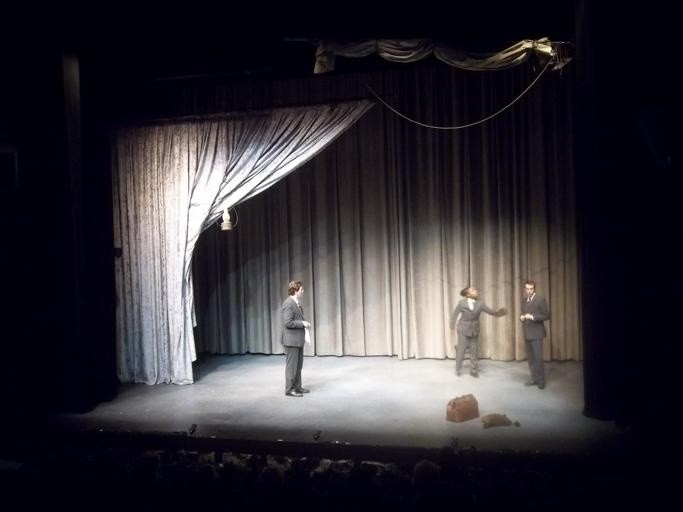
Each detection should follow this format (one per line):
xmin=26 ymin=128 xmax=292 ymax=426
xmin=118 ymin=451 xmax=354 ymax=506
xmin=518 ymin=279 xmax=552 ymax=391
xmin=447 ymin=286 xmax=510 ymax=380
xmin=276 ymin=280 xmax=314 ymax=398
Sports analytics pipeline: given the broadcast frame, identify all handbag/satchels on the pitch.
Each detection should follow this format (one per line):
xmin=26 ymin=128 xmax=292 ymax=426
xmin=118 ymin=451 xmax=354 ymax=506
xmin=483 ymin=414 xmax=511 ymax=426
xmin=446 ymin=395 xmax=479 ymax=422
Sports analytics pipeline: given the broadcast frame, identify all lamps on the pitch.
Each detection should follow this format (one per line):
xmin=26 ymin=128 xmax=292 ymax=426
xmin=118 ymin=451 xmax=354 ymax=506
xmin=530 ymin=38 xmax=571 ymax=73
xmin=219 ymin=207 xmax=232 ymax=232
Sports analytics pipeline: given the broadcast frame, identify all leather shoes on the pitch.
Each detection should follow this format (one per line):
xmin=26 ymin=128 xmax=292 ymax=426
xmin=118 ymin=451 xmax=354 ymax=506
xmin=471 ymin=369 xmax=480 ymax=379
xmin=287 ymin=388 xmax=309 ymax=396
xmin=539 ymin=383 xmax=545 ymax=390
xmin=525 ymin=378 xmax=535 ymax=387
